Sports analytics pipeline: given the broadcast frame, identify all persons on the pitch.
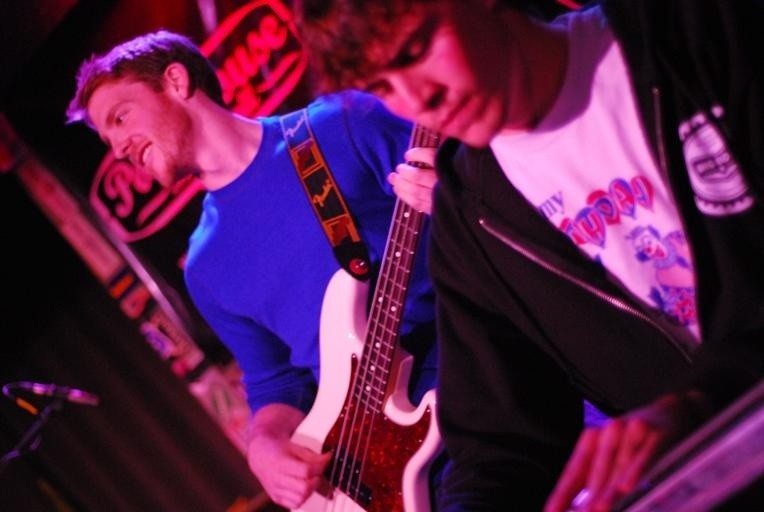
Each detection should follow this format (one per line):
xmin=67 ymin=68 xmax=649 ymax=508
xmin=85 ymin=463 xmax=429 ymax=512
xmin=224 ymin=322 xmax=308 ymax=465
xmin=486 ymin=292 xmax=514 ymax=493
xmin=303 ymin=1 xmax=764 ymax=509
xmin=63 ymin=28 xmax=444 ymax=512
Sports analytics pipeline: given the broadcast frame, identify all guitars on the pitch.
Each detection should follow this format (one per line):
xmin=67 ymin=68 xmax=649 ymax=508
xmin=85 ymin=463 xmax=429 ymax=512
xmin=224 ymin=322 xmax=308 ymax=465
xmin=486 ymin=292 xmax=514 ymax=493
xmin=289 ymin=125 xmax=440 ymax=512
